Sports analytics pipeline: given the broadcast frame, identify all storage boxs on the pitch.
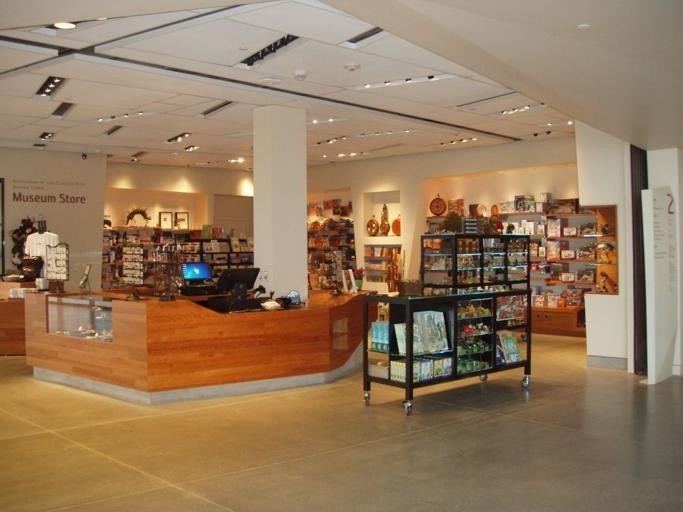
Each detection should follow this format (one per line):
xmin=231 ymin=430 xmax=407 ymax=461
xmin=548 ymin=198 xmax=579 ymax=215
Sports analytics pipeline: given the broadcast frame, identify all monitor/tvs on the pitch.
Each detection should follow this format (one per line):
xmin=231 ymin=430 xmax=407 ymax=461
xmin=217 ymin=268 xmax=260 ymax=300
xmin=181 ymin=262 xmax=212 ymax=285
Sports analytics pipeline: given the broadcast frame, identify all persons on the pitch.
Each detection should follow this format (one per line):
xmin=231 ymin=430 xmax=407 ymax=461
xmin=11 ymin=219 xmax=60 ymax=278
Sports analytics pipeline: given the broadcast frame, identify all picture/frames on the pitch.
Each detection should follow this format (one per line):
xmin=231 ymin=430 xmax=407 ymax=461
xmin=159 ymin=211 xmax=189 ymax=230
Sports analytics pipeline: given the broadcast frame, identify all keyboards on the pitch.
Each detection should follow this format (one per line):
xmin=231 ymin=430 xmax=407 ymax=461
xmin=181 ymin=286 xmax=216 ymax=295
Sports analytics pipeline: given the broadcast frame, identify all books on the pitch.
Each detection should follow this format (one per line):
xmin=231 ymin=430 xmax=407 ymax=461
xmin=497 ymin=332 xmax=521 ymax=363
xmin=389 ymin=311 xmax=452 ymax=383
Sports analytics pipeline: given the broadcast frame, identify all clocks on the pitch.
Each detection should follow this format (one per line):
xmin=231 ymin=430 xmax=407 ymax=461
xmin=429 ymin=198 xmax=446 ymax=216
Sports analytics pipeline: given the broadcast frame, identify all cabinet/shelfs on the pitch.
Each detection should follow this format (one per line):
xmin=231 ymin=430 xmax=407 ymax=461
xmin=421 ymin=206 xmax=619 ymax=337
xmin=102 ymin=227 xmax=253 ymax=289
xmin=362 ymin=232 xmax=532 ymax=416
